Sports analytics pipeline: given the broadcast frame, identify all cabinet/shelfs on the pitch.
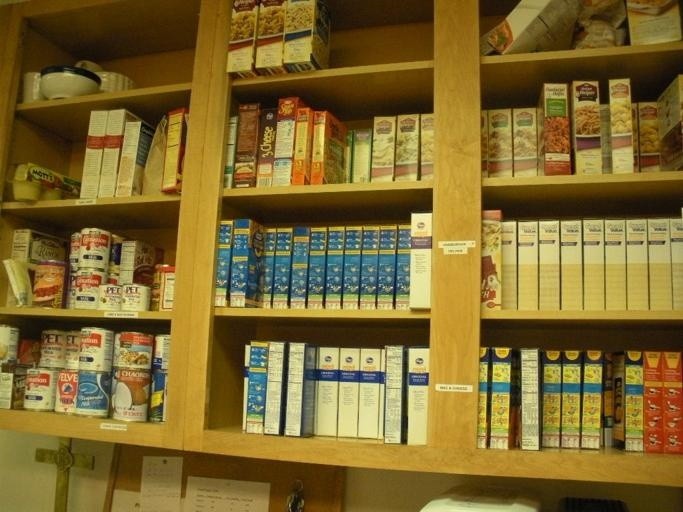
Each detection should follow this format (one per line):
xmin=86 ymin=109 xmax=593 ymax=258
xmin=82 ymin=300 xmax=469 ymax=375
xmin=1 ymin=0 xmax=683 ymax=512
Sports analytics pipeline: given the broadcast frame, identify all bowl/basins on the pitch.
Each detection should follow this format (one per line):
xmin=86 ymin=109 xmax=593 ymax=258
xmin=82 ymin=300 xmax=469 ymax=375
xmin=41 ymin=66 xmax=101 ymax=99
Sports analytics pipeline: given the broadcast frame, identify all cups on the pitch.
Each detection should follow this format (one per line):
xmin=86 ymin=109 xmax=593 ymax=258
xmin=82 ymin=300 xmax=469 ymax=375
xmin=23 ymin=71 xmax=40 ymax=101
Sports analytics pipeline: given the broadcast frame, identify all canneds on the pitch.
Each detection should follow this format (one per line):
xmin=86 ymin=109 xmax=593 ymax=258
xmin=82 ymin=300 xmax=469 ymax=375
xmin=23 ymin=227 xmax=175 ymax=422
